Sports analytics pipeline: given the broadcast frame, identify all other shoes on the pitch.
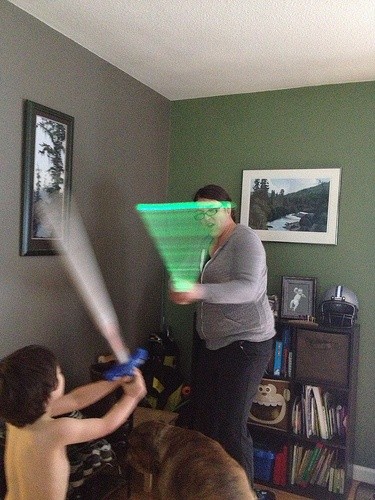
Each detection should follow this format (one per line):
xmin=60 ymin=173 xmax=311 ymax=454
xmin=79 ymin=452 xmax=92 ymax=476
xmin=98 ymin=443 xmax=112 ymax=462
xmin=70 ymin=461 xmax=84 ymax=488
xmin=86 ymin=449 xmax=101 ymax=466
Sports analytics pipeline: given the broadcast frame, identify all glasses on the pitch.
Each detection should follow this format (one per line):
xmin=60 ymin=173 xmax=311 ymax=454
xmin=194 ymin=207 xmax=223 ymax=222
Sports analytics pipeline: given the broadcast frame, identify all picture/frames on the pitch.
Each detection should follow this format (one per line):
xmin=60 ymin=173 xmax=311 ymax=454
xmin=240 ymin=168 xmax=341 ymax=245
xmin=281 ymin=276 xmax=316 ymax=321
xmin=20 ymin=99 xmax=75 ymax=257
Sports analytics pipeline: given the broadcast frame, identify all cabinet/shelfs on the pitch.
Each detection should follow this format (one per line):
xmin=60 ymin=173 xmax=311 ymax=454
xmin=190 ymin=312 xmax=360 ymax=500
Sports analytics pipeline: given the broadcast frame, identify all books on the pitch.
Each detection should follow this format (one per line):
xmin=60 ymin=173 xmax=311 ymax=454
xmin=291 ymin=442 xmax=344 ymax=495
xmin=291 ymin=385 xmax=348 ymax=442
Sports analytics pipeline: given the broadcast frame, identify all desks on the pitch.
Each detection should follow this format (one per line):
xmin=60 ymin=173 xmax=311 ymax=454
xmin=88 ymin=355 xmax=183 ymax=411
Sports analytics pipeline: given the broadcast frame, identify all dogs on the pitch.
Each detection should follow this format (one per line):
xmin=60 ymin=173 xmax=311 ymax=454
xmin=126 ymin=420 xmax=257 ymax=499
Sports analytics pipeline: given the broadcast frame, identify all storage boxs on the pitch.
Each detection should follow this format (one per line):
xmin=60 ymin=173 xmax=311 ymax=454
xmin=253 ymin=448 xmax=276 ymax=482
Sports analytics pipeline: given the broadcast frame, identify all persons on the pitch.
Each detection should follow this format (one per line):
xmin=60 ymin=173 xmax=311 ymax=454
xmin=289 ymin=286 xmax=307 ymax=309
xmin=0 ymin=344 xmax=148 ymax=500
xmin=167 ymin=184 xmax=277 ymax=488
